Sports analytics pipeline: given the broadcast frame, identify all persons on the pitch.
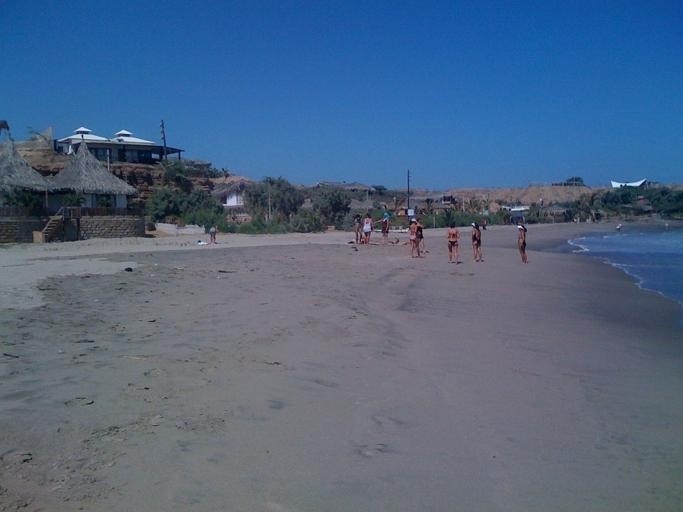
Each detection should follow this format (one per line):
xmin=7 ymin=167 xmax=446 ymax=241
xmin=210 ymin=222 xmax=219 ymax=243
xmin=518 ymin=224 xmax=528 ymax=265
xmin=352 ymin=211 xmax=487 ymax=264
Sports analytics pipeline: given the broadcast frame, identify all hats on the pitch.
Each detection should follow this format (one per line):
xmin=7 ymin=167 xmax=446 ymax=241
xmin=518 ymin=226 xmax=524 ymax=229
xmin=411 ymin=219 xmax=417 ymax=224
xmin=472 ymin=223 xmax=475 ymax=227
